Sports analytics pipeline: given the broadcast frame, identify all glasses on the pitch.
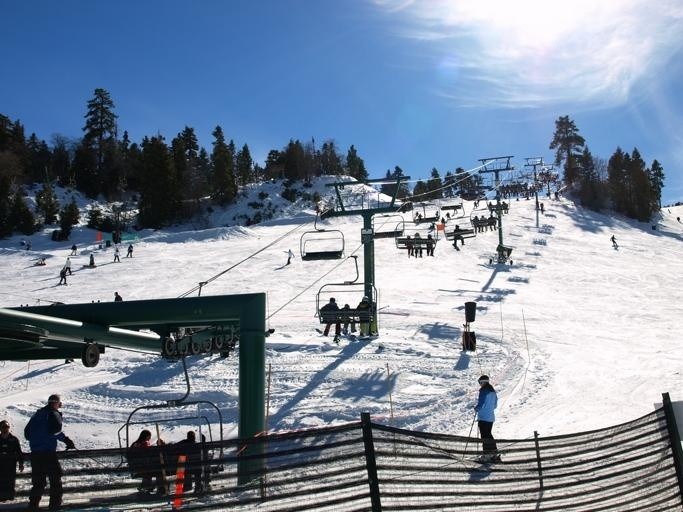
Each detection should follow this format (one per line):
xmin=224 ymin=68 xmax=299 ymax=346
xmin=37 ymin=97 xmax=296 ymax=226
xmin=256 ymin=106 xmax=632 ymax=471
xmin=58 ymin=402 xmax=61 ymax=408
xmin=479 ymin=382 xmax=486 ymax=384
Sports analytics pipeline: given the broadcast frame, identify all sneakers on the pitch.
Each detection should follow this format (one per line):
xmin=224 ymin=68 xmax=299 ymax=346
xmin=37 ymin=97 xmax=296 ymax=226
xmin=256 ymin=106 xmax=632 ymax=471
xmin=491 ymin=455 xmax=501 ymax=461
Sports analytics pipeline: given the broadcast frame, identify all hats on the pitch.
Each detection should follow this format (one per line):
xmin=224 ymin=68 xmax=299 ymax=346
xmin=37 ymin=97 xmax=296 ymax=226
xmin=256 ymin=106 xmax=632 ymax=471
xmin=478 ymin=375 xmax=489 ymax=383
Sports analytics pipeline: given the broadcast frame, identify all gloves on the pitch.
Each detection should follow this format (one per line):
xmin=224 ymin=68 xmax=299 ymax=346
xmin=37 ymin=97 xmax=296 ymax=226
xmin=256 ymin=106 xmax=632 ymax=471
xmin=62 ymin=437 xmax=73 ymax=449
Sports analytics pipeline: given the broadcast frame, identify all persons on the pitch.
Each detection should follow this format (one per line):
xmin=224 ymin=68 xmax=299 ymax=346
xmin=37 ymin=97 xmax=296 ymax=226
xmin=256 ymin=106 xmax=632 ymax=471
xmin=405 ymin=178 xmax=544 ymax=258
xmin=20 ymin=234 xmax=137 ymax=286
xmin=128 ymin=428 xmax=213 ymax=494
xmin=0 ymin=420 xmax=25 ymax=502
xmin=25 ymin=393 xmax=74 ymax=511
xmin=283 ymin=247 xmax=295 ymax=265
xmin=320 ymin=297 xmax=372 ymax=341
xmin=472 ymin=372 xmax=499 ymax=461
xmin=610 ymin=234 xmax=617 ymax=246
